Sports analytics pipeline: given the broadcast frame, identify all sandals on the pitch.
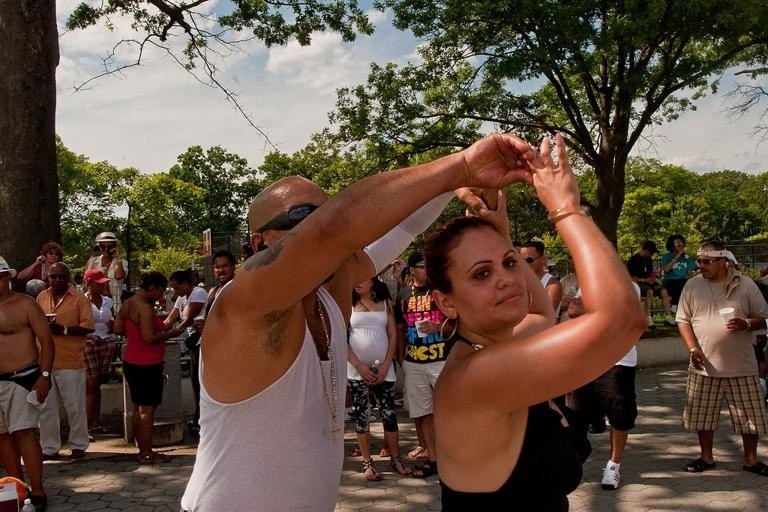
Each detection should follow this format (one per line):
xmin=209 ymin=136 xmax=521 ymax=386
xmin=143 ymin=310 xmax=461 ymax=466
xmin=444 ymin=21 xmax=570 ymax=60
xmin=391 ymin=447 xmax=437 ymax=478
xmin=362 ymin=459 xmax=380 ymax=481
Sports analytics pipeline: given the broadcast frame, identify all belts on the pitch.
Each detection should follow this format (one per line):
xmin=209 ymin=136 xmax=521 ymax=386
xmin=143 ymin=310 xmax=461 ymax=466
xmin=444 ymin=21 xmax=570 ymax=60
xmin=0 ymin=363 xmax=40 ymax=377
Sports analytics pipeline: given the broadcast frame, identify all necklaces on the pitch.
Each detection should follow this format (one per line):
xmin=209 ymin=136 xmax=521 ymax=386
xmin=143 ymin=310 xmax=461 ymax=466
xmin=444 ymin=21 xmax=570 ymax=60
xmin=453 ymin=332 xmax=488 ymax=353
xmin=303 ymin=298 xmax=339 ymax=418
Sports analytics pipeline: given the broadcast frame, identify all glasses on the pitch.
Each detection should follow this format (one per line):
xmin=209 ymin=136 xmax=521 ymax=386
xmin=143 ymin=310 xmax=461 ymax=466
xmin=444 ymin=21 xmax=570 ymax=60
xmin=50 ymin=274 xmax=66 ymax=279
xmin=525 ymin=255 xmax=541 ymax=263
xmin=698 ymin=259 xmax=721 ymax=264
xmin=256 ymin=206 xmax=319 ymax=230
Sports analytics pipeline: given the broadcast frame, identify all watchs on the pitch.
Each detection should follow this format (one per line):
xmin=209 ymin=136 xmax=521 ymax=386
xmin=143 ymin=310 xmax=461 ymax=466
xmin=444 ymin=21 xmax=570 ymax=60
xmin=40 ymin=371 xmax=51 ymax=377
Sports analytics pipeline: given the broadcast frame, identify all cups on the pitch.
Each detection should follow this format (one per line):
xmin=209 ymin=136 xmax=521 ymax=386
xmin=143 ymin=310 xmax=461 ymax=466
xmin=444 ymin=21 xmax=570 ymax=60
xmin=649 ymin=271 xmax=658 ymax=283
xmin=27 ymin=388 xmax=45 ymax=410
xmin=415 ymin=319 xmax=431 ymax=339
xmin=45 ymin=313 xmax=56 ymax=326
xmin=720 ymin=306 xmax=735 ymax=331
xmin=194 ymin=314 xmax=205 ymax=327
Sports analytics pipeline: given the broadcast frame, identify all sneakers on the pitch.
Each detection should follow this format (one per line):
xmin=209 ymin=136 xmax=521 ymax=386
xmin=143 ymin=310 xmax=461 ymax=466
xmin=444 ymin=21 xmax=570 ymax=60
xmin=664 ymin=317 xmax=677 ymax=326
xmin=71 ymin=449 xmax=84 ymax=458
xmin=41 ymin=452 xmax=59 ymax=459
xmin=137 ymin=452 xmax=170 ymax=463
xmin=649 ymin=318 xmax=655 ymax=327
xmin=30 ymin=495 xmax=47 ymax=512
xmin=602 ymin=465 xmax=621 ymax=489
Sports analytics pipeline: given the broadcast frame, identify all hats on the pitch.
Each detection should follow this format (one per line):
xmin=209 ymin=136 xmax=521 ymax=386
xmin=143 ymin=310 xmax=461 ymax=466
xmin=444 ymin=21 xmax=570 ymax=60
xmin=84 ymin=269 xmax=109 ymax=283
xmin=408 ymin=252 xmax=423 ymax=265
xmin=0 ymin=256 xmax=17 ymax=277
xmin=643 ymin=240 xmax=659 ymax=253
xmin=95 ymin=232 xmax=118 ymax=241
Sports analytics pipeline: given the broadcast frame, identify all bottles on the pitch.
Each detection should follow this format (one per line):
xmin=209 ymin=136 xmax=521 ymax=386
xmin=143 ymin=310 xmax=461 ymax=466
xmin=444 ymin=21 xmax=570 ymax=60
xmin=365 ymin=359 xmax=381 ymax=383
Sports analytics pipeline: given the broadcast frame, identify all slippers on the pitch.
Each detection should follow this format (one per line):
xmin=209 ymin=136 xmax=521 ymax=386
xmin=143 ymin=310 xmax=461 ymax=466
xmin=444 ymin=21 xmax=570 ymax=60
xmin=743 ymin=461 xmax=768 ymax=476
xmin=682 ymin=458 xmax=715 ymax=472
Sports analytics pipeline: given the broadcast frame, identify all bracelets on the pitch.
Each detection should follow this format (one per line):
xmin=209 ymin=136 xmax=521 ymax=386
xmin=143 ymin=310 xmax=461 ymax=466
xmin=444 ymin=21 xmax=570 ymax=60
xmin=548 ymin=206 xmax=592 ymax=224
xmin=746 ymin=319 xmax=751 ymax=328
xmin=690 ymin=348 xmax=700 ymax=352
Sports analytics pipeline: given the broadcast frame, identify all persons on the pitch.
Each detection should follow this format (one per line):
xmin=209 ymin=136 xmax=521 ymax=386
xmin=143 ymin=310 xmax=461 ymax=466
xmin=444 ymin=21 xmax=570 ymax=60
xmin=517 ymin=241 xmax=641 ymax=490
xmin=662 ymin=234 xmax=697 ymax=314
xmin=674 ymin=240 xmax=767 ymax=476
xmin=2 ymin=257 xmax=55 ymax=512
xmin=427 ymin=133 xmax=650 ymax=511
xmin=18 ymin=232 xmax=254 ymax=465
xmin=757 ymin=269 xmax=767 ymax=285
xmin=346 ymin=250 xmax=461 ymax=480
xmin=179 ymin=132 xmax=534 ymax=511
xmin=628 ymin=240 xmax=677 ymax=327
